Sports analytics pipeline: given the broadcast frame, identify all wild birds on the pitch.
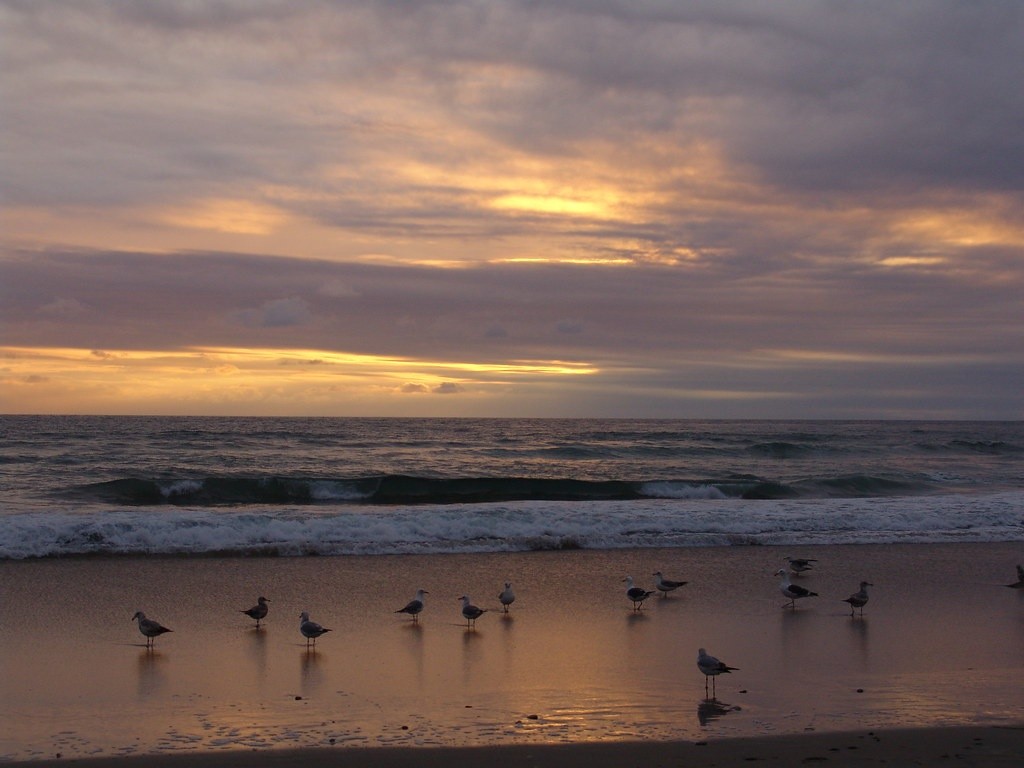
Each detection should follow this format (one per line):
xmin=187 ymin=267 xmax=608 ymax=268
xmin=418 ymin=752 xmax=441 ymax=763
xmin=499 ymin=582 xmax=515 ymax=612
xmin=458 ymin=596 xmax=487 ymax=625
xmin=623 ymin=576 xmax=656 ymax=610
xmin=1015 ymin=564 xmax=1024 ymax=582
xmin=131 ymin=612 xmax=174 ymax=646
xmin=842 ymin=581 xmax=875 ymax=615
xmin=775 ymin=568 xmax=818 ymax=610
xmin=299 ymin=611 xmax=333 ymax=644
xmin=394 ymin=589 xmax=429 ymax=621
xmin=696 ymin=647 xmax=740 ymax=682
xmin=784 ymin=555 xmax=817 ymax=575
xmin=239 ymin=596 xmax=272 ymax=627
xmin=653 ymin=572 xmax=688 ymax=598
